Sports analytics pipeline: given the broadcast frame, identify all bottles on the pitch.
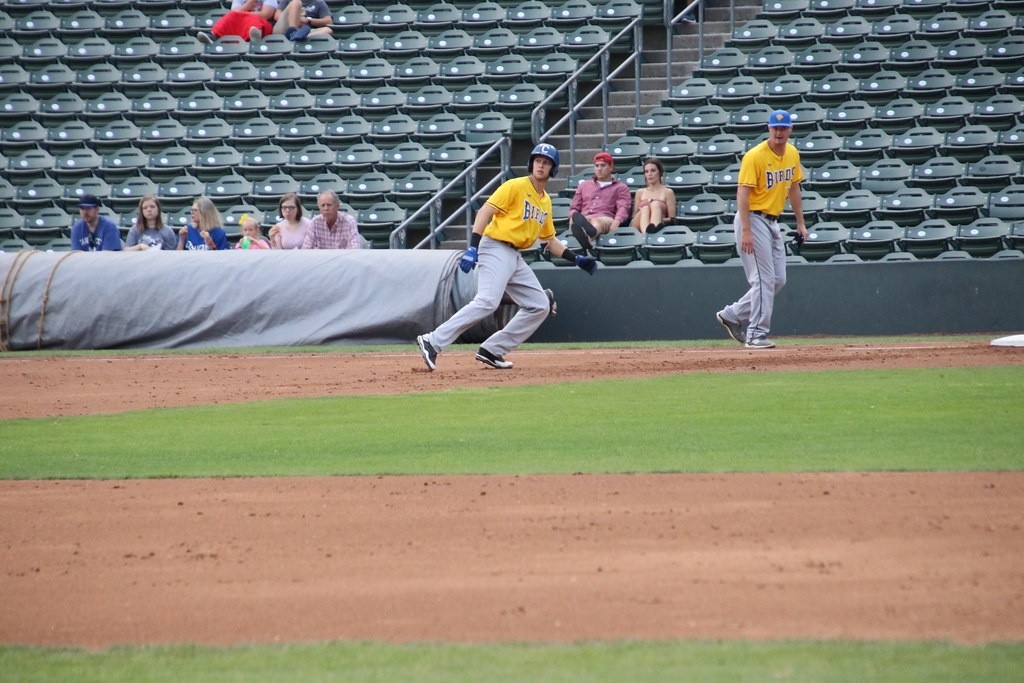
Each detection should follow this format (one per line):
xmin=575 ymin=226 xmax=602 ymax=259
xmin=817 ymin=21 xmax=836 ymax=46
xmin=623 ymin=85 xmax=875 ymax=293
xmin=242 ymin=236 xmax=251 ymax=249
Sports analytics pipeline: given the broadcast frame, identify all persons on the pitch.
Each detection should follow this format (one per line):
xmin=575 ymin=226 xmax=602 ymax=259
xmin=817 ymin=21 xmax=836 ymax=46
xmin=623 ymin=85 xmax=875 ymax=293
xmin=569 ymin=153 xmax=631 ymax=251
xmin=197 ymin=0 xmax=333 ymax=45
xmin=123 ymin=194 xmax=178 ymax=252
xmin=71 ymin=194 xmax=122 ymax=252
xmin=630 ymin=158 xmax=677 ymax=235
xmin=417 ymin=143 xmax=598 ymax=371
xmin=235 ymin=190 xmax=362 ymax=250
xmin=717 ymin=109 xmax=808 ymax=350
xmin=176 ymin=197 xmax=232 ymax=250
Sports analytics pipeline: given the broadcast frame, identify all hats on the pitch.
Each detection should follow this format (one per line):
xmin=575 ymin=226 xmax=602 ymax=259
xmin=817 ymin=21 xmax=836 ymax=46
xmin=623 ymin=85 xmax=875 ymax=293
xmin=768 ymin=109 xmax=791 ymax=127
xmin=75 ymin=194 xmax=98 ymax=207
xmin=593 ymin=153 xmax=612 ymax=165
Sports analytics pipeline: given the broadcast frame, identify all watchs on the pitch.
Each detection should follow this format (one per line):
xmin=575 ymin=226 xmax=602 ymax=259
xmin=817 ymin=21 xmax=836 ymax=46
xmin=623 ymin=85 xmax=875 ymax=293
xmin=307 ymin=16 xmax=312 ymax=26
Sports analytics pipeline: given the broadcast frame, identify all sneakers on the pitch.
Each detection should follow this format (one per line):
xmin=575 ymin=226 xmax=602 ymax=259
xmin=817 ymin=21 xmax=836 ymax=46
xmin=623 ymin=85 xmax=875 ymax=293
xmin=475 ymin=347 xmax=514 ymax=368
xmin=745 ymin=336 xmax=776 ymax=348
xmin=417 ymin=334 xmax=437 ymax=371
xmin=716 ymin=311 xmax=745 ymax=344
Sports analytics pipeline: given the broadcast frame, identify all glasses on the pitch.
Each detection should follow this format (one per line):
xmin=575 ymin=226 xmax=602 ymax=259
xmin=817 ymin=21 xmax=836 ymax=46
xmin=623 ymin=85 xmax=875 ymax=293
xmin=189 ymin=209 xmax=198 ymax=214
xmin=281 ymin=205 xmax=295 ymax=211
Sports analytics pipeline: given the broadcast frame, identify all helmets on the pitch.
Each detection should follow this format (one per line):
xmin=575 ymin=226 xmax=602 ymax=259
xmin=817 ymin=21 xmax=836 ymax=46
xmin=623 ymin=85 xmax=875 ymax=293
xmin=526 ymin=143 xmax=560 ymax=177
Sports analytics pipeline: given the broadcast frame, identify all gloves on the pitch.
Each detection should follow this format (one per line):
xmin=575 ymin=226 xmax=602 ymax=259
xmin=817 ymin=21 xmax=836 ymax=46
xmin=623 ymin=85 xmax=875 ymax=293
xmin=574 ymin=254 xmax=598 ymax=275
xmin=786 ymin=231 xmax=804 ymax=255
xmin=460 ymin=247 xmax=478 ymax=274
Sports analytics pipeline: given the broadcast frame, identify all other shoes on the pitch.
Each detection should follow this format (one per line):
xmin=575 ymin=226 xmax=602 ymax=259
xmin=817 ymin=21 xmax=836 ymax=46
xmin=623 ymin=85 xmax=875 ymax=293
xmin=248 ymin=26 xmax=262 ymax=42
xmin=197 ymin=31 xmax=216 ymax=44
xmin=645 ymin=223 xmax=664 ymax=234
xmin=284 ymin=25 xmax=311 ymax=42
xmin=571 ymin=212 xmax=597 ymax=249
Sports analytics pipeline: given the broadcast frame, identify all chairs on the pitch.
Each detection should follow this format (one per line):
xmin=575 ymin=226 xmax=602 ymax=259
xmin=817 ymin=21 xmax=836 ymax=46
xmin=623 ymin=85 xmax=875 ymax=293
xmin=0 ymin=0 xmax=1024 ymax=266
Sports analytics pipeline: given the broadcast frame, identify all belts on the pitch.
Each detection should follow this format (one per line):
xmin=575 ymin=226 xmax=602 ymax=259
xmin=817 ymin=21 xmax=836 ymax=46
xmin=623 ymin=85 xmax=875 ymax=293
xmin=755 ymin=211 xmax=778 ymax=222
xmin=501 ymin=241 xmax=519 ymax=251
